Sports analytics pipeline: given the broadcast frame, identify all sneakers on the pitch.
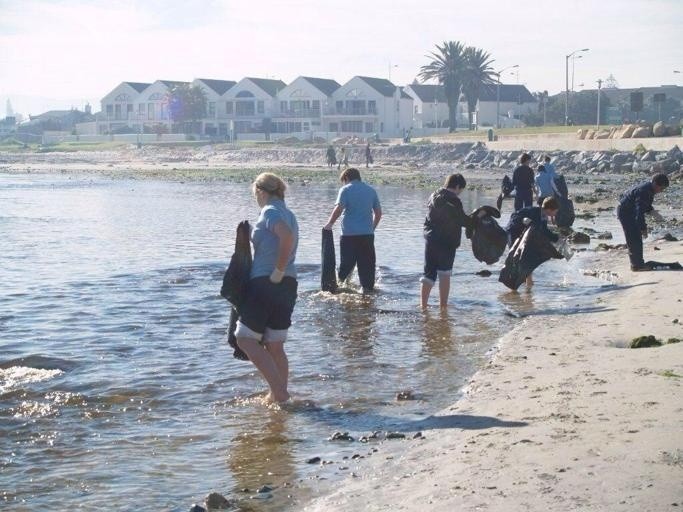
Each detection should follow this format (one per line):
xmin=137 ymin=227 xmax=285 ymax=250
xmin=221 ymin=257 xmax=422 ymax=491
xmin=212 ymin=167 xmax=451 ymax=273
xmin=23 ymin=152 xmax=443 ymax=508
xmin=631 ymin=262 xmax=651 ymax=271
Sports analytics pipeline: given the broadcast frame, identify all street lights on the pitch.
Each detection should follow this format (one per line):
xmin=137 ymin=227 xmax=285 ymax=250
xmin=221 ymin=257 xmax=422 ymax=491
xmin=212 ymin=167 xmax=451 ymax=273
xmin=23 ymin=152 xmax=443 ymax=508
xmin=496 ymin=65 xmax=519 ymax=128
xmin=595 ymin=79 xmax=603 ymax=131
xmin=564 ymin=49 xmax=589 ymax=126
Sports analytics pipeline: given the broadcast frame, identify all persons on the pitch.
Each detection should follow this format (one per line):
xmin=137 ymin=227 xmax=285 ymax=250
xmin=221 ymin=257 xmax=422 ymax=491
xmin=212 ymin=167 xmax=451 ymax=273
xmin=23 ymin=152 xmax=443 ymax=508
xmin=503 ymin=154 xmax=536 ymax=211
xmin=404 ymin=130 xmax=408 ymax=143
xmin=340 ymin=146 xmax=348 ymax=168
xmin=542 ymin=157 xmax=560 ymax=180
xmin=420 ymin=174 xmax=488 ymax=314
xmin=365 ymin=143 xmax=372 ymax=168
xmin=326 ymin=144 xmax=336 ymax=167
xmin=535 ymin=165 xmax=562 ymax=225
xmin=501 ymin=198 xmax=559 ymax=301
xmin=617 ymin=173 xmax=669 ymax=271
xmin=408 ymin=125 xmax=413 ymax=143
xmin=234 ymin=172 xmax=300 ymax=406
xmin=323 ymin=168 xmax=382 ymax=294
